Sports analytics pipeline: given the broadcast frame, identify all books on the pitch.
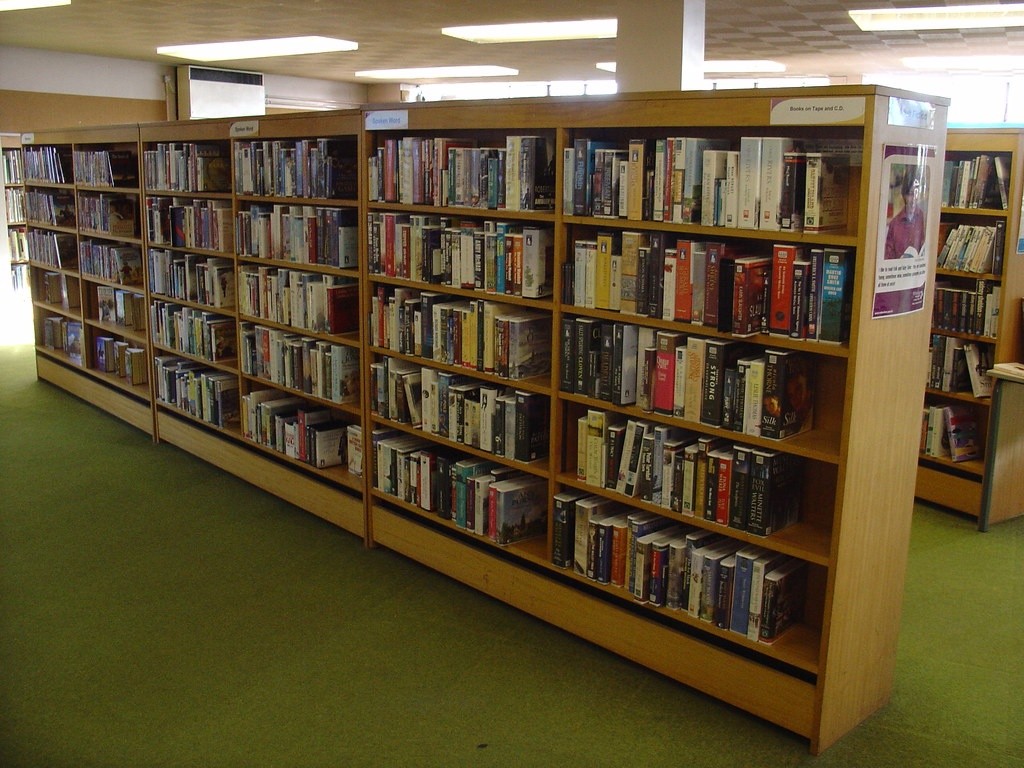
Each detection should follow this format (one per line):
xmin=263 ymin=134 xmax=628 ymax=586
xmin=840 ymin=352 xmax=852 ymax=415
xmin=920 ymin=154 xmax=1013 ymax=465
xmin=0 ymin=136 xmax=859 ymax=649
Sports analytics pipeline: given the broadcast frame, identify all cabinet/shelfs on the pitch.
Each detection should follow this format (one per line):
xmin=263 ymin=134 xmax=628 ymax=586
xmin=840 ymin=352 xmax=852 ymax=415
xmin=0 ymin=123 xmax=156 ymax=443
xmin=361 ymin=85 xmax=952 ymax=756
xmin=138 ymin=107 xmax=369 ymax=550
xmin=913 ymin=127 xmax=1024 ymax=533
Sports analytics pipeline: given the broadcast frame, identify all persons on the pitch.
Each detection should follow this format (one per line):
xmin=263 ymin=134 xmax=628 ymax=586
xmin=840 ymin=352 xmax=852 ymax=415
xmin=884 ymin=166 xmax=925 ymax=259
